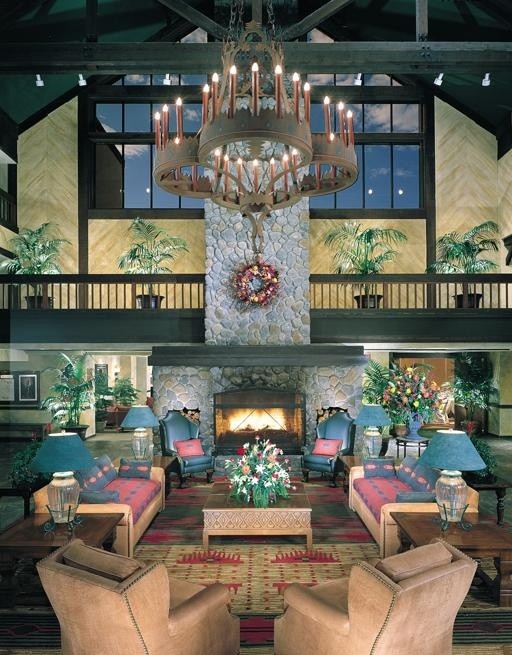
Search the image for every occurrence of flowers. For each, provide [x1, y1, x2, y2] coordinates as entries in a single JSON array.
[[224, 435, 297, 509]]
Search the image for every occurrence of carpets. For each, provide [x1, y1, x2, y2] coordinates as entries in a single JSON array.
[[0, 477, 511, 655]]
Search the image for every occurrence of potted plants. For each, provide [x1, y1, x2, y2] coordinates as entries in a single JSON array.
[[361, 352, 498, 457], [39, 350, 142, 440]]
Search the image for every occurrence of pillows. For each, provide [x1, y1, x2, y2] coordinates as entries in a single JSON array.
[[74, 454, 152, 504], [174, 438, 204, 457], [312, 439, 342, 456], [363, 454, 441, 503]]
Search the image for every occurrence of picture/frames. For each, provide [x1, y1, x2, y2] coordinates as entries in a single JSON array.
[[0, 370, 38, 403]]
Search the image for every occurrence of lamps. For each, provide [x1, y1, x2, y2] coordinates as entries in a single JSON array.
[[30, 430, 96, 533], [416, 428, 487, 532], [120, 405, 160, 459]]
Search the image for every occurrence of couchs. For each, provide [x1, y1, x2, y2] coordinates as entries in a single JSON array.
[[349, 454, 480, 559], [33, 454, 166, 557]]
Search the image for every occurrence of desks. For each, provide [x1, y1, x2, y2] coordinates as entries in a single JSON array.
[[0, 423, 50, 442]]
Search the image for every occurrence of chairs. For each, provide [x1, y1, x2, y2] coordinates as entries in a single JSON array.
[[300, 412, 356, 488], [159, 410, 216, 488]]
